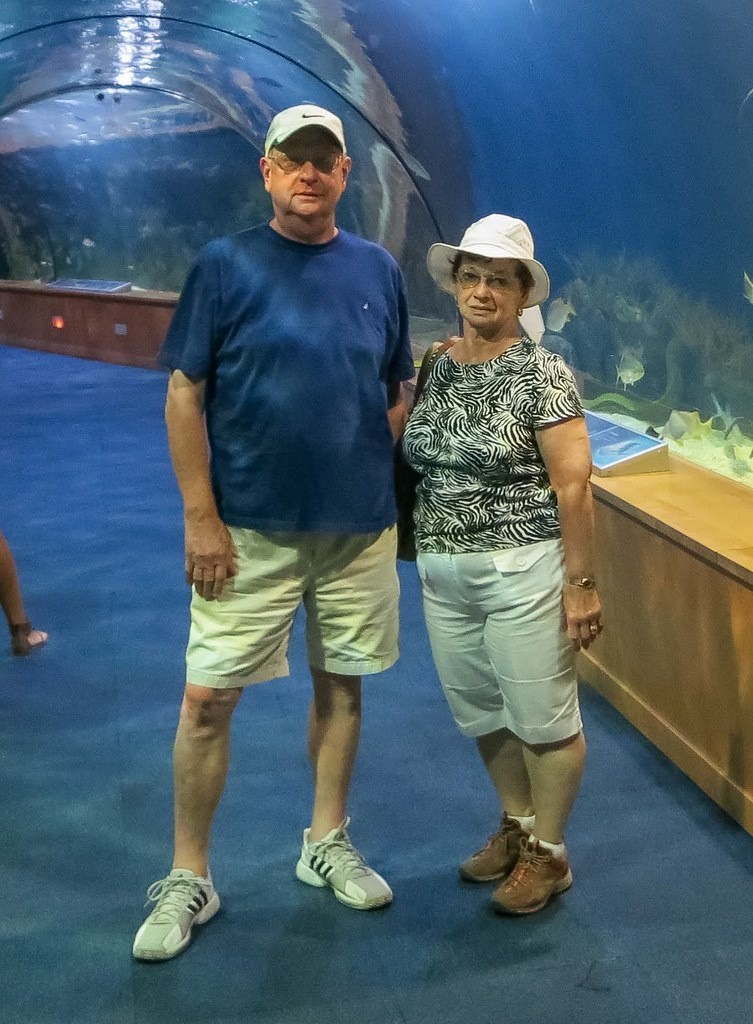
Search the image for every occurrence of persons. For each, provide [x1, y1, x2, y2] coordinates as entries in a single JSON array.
[[0, 534, 48, 656], [132, 104, 415, 961], [398, 214, 604, 914]]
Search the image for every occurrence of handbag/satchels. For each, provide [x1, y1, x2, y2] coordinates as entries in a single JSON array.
[[393, 447, 424, 562]]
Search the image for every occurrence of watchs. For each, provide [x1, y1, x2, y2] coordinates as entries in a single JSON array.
[[564, 575, 596, 590]]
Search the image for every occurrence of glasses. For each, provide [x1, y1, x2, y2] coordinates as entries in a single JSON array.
[[454, 265, 522, 291], [268, 151, 341, 172]]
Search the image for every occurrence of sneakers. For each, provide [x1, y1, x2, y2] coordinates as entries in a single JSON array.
[[459, 810, 531, 882], [133, 864, 220, 961], [490, 837, 573, 913], [296, 817, 393, 910]]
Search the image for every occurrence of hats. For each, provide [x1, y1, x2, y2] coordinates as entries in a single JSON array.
[[426, 214, 550, 309], [265, 104, 347, 158]]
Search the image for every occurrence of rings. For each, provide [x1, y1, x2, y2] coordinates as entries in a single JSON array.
[[590, 625, 598, 631]]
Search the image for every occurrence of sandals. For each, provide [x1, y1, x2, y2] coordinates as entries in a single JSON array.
[[8, 620, 48, 655]]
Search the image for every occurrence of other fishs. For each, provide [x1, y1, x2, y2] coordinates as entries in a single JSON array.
[[545, 297, 577, 334], [615, 352, 646, 391], [253, 75, 283, 88], [662, 410, 753, 478], [743, 270, 753, 306]]
